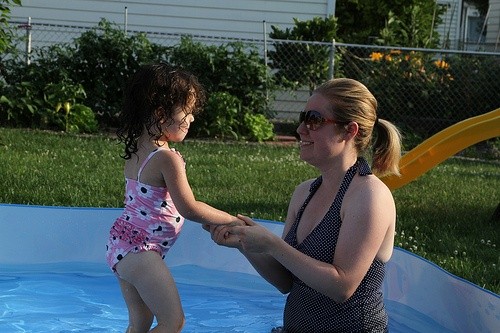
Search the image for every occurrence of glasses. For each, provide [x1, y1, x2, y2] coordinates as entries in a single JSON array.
[[300, 110, 362, 137]]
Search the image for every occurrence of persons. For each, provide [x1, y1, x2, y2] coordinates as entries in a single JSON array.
[[205, 76, 407, 333], [105, 63, 246, 333]]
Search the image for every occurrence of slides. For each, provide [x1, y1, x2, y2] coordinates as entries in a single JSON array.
[[374, 108, 499, 193]]
